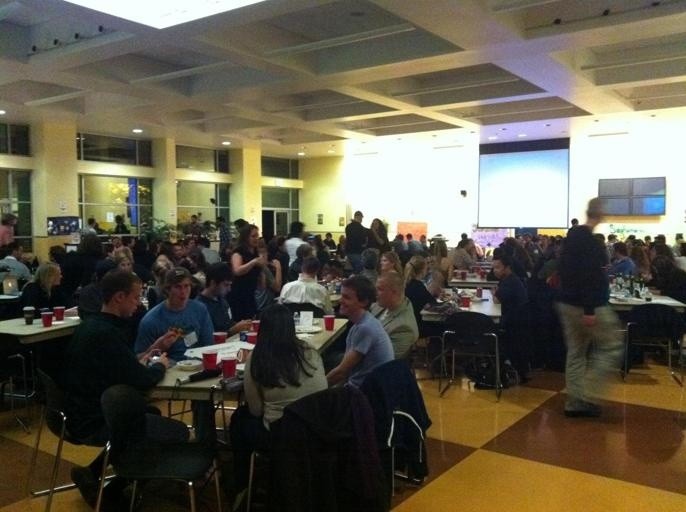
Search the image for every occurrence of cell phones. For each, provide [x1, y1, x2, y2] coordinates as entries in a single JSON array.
[[147, 354, 160, 368]]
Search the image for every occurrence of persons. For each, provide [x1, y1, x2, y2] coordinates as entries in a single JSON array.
[[554, 198, 624, 415], [487, 219, 563, 385], [595, 232, 685, 374], [43, 261, 420, 512], [2, 211, 483, 394]]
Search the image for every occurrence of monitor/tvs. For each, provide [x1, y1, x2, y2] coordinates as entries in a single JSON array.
[[47, 216, 79, 235]]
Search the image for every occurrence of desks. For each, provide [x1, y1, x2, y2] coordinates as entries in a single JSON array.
[[142, 315, 348, 441], [1, 308, 96, 402]]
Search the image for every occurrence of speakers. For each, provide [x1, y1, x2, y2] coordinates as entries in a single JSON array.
[[632, 178, 665, 197], [599, 198, 632, 216], [632, 196, 665, 216], [599, 179, 632, 197]]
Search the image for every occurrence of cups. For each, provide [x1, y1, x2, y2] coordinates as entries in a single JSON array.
[[251, 320, 260, 332], [245, 332, 257, 343], [39, 307, 49, 313], [324, 279, 346, 294], [22, 306, 35, 325], [608, 274, 614, 283], [623, 274, 632, 289], [221, 356, 236, 378], [11, 288, 19, 296], [201, 349, 217, 369], [150, 348, 164, 365], [451, 269, 486, 307], [322, 315, 336, 331], [213, 332, 227, 344], [40, 312, 54, 327], [52, 306, 66, 320]]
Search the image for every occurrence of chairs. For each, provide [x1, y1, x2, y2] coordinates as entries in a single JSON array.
[[365, 355, 424, 502], [0, 344, 44, 433], [28, 368, 108, 510], [255, 386, 378, 511], [621, 302, 685, 387], [98, 387, 223, 509], [436, 311, 521, 399]]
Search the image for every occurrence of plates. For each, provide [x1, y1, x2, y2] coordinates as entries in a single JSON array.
[[234, 362, 247, 372], [176, 360, 201, 371], [294, 326, 321, 334]]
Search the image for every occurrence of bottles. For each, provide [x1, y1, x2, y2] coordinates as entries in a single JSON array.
[[633, 272, 652, 301], [292, 312, 298, 325], [615, 272, 622, 290]]
